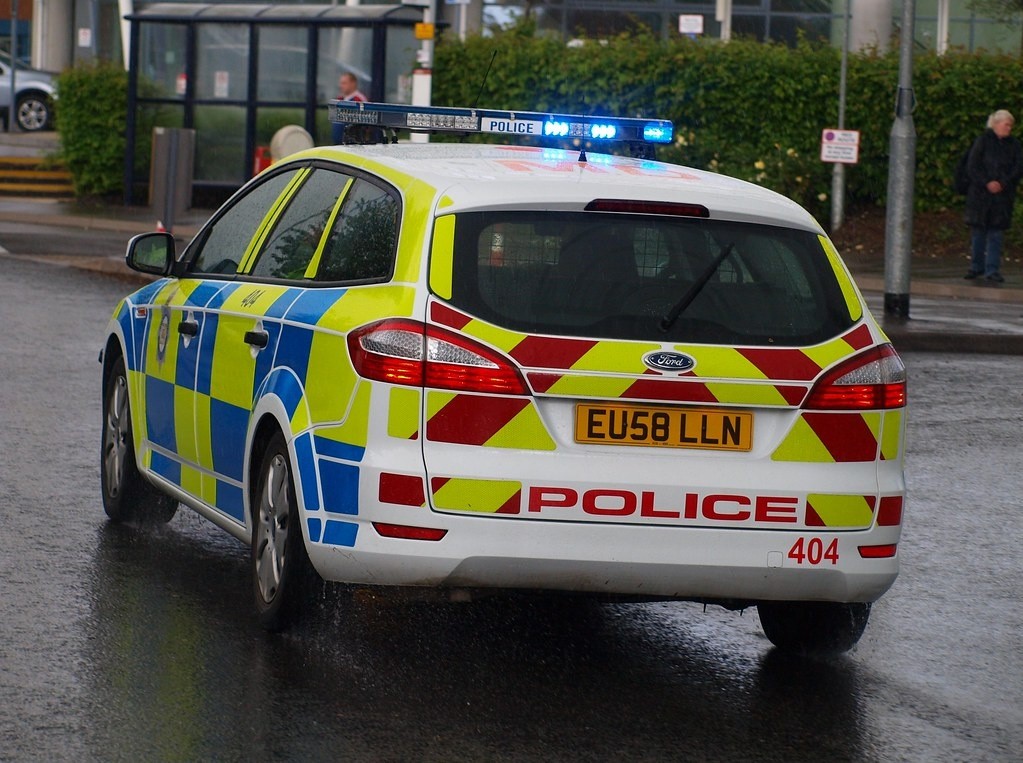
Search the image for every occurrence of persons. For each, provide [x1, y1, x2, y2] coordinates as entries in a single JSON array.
[[963, 109, 1020, 283], [332, 73, 378, 145]]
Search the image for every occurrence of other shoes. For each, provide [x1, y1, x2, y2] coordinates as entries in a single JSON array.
[[963, 268, 984, 280], [987, 272, 1004, 282]]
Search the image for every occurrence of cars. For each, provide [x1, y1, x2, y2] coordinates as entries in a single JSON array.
[[101, 101, 909, 666], [0, 50, 62, 134]]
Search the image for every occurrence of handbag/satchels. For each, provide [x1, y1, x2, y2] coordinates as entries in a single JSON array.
[[952, 158, 975, 194]]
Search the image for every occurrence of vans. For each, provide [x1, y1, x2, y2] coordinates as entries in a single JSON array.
[[173, 45, 373, 105]]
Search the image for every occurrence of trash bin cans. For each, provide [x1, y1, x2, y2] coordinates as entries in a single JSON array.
[[149, 126, 196, 232]]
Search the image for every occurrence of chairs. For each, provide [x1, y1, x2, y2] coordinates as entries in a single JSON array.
[[550, 224, 641, 316]]
